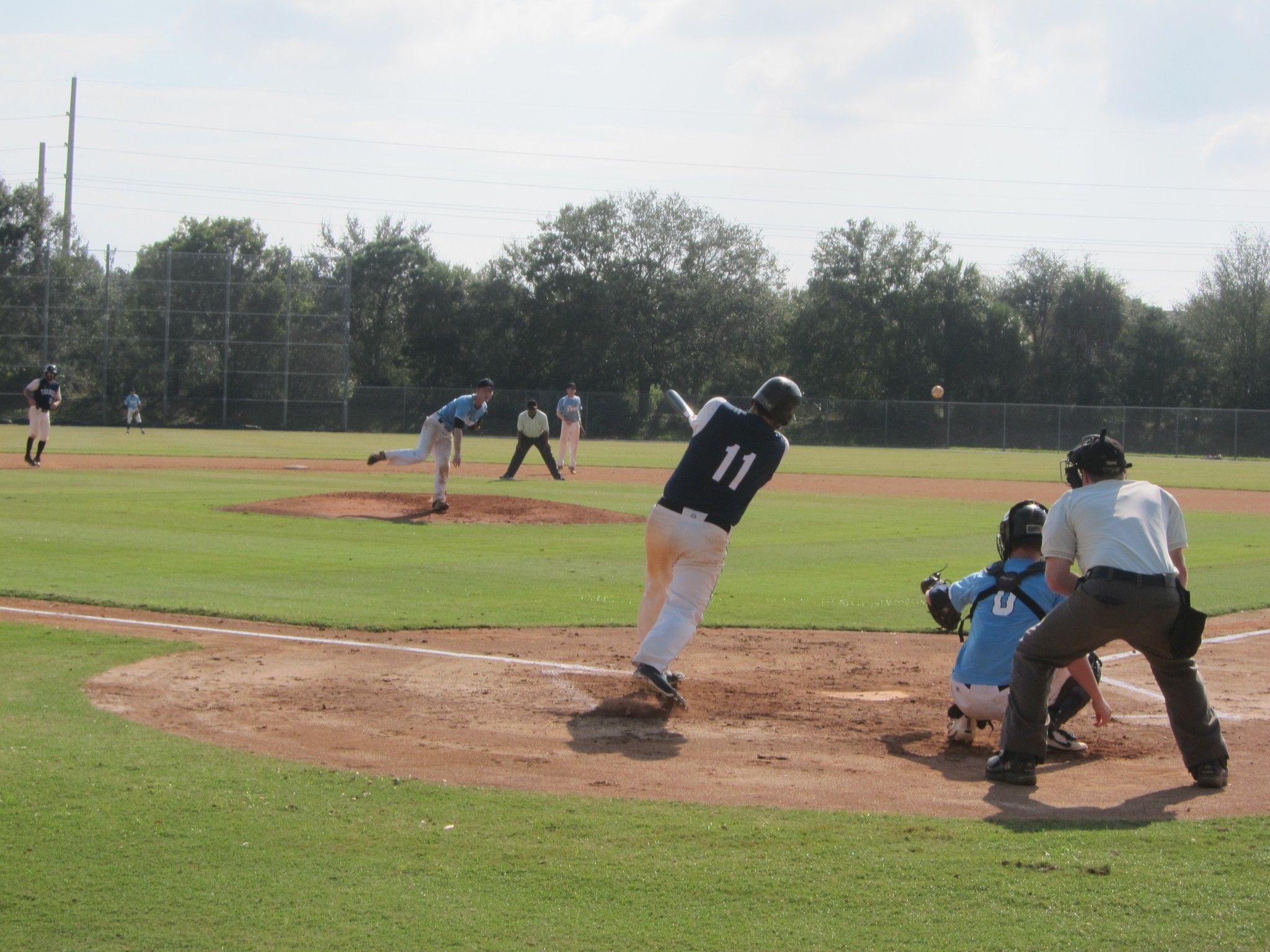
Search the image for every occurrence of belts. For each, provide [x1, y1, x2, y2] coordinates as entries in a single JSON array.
[[36, 406, 49, 414], [438, 418, 451, 432], [1085, 565, 1177, 588], [964, 684, 1009, 691], [658, 498, 731, 533]]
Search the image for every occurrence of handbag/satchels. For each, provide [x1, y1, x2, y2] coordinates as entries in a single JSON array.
[[1169, 578, 1208, 659]]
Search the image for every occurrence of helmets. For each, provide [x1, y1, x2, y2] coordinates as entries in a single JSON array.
[[752, 376, 803, 420], [996, 499, 1049, 562], [44, 364, 57, 375], [1065, 428, 1132, 490]]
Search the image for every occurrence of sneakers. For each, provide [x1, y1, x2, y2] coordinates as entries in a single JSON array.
[[367, 449, 384, 465], [986, 756, 1037, 785], [664, 669, 684, 684], [632, 663, 689, 712], [1197, 762, 1228, 787], [947, 714, 977, 744], [433, 500, 449, 512], [1047, 729, 1088, 751]]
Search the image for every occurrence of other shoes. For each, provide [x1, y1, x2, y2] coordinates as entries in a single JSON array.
[[33, 457, 40, 467], [499, 475, 513, 479], [568, 466, 576, 474], [25, 456, 35, 466], [555, 476, 566, 480], [558, 465, 563, 474]]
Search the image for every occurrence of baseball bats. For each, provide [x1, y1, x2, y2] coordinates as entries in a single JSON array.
[[664, 389, 694, 419]]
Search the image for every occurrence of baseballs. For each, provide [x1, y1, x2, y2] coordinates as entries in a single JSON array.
[[932, 386, 944, 398]]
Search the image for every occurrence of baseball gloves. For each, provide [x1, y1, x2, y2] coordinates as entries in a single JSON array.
[[468, 410, 488, 431], [920, 577, 961, 630], [579, 426, 586, 439]]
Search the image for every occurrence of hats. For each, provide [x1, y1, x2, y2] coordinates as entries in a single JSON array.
[[567, 382, 576, 388], [527, 400, 537, 409], [478, 378, 495, 389]]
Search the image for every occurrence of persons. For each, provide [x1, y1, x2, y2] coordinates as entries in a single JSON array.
[[556, 382, 582, 474], [24, 364, 62, 466], [631, 377, 802, 697], [499, 400, 566, 480], [986, 426, 1230, 788], [368, 378, 494, 510], [920, 500, 1110, 750], [125, 388, 145, 434]]
